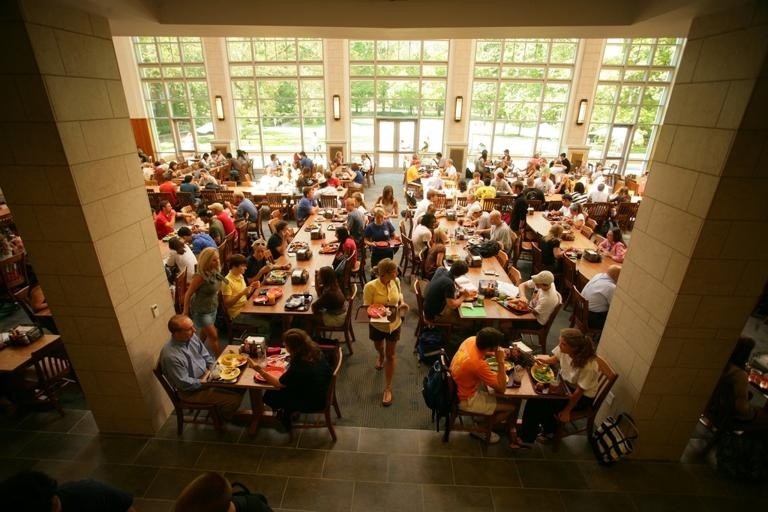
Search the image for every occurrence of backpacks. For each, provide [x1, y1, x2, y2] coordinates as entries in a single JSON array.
[[421, 360, 457, 416]]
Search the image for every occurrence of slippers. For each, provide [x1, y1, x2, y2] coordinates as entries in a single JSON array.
[[509, 437, 533, 448]]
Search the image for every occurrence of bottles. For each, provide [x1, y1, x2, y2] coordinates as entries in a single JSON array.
[[512, 343, 518, 362], [535, 382, 544, 394], [265, 346, 282, 355], [8, 330, 30, 344], [486, 282, 495, 298], [241, 340, 261, 357]]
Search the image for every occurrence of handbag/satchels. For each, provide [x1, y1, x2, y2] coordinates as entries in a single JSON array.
[[445, 335, 467, 364], [419, 329, 445, 366], [591, 412, 640, 466], [463, 241, 499, 257]]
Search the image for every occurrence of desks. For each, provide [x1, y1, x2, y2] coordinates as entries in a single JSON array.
[[200, 345, 294, 429], [489, 354, 572, 440], [1, 329, 61, 393], [508, 177, 528, 186], [158, 216, 207, 268]]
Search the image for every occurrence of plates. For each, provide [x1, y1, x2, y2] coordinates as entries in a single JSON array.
[[490, 361, 514, 372], [377, 239, 402, 247], [367, 304, 386, 318], [210, 353, 247, 381], [163, 217, 208, 242], [265, 211, 346, 284], [456, 290, 478, 302], [255, 354, 289, 382], [251, 295, 268, 303], [267, 287, 282, 298], [506, 299, 530, 312], [286, 299, 301, 308], [531, 363, 554, 385], [434, 207, 485, 265]]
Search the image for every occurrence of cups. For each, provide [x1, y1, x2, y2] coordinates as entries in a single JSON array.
[[499, 292, 505, 302], [512, 368, 525, 385], [303, 289, 310, 299], [547, 377, 560, 392], [577, 252, 582, 260], [255, 281, 260, 288], [269, 293, 275, 303], [258, 350, 267, 368], [477, 295, 484, 305], [259, 290, 266, 295]]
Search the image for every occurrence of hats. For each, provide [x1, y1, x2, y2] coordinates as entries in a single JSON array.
[[469, 204, 481, 213], [531, 270, 555, 284], [207, 203, 223, 212], [169, 471, 232, 512]]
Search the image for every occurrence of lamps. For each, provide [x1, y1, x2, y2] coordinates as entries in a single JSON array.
[[576, 99, 588, 125], [453, 96, 463, 121], [215, 95, 224, 120], [332, 94, 340, 120]]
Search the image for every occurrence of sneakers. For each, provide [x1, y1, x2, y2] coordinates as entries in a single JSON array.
[[470, 423, 500, 443], [376, 354, 384, 369], [382, 386, 393, 406]]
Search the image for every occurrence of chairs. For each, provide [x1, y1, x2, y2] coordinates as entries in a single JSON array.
[[221, 204, 373, 346], [1, 252, 44, 327], [439, 347, 496, 449], [405, 158, 461, 199], [625, 174, 639, 195], [309, 159, 376, 208], [144, 179, 354, 219], [575, 161, 617, 185], [153, 361, 227, 445], [171, 154, 253, 179], [288, 347, 344, 441], [174, 204, 265, 314], [30, 338, 82, 419], [545, 353, 620, 451], [399, 195, 639, 368], [702, 377, 756, 457]]
